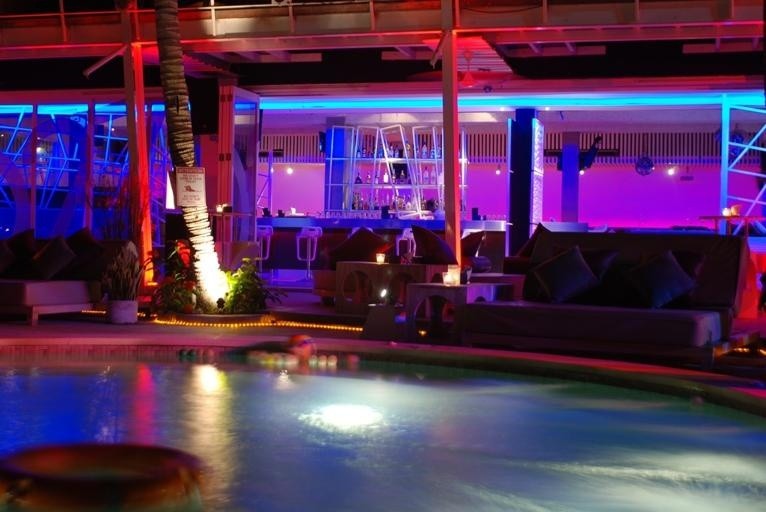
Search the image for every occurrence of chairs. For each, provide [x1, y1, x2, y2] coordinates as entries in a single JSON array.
[[351, 226, 373, 235], [254, 224, 273, 280], [396, 228, 416, 257], [296, 226, 322, 281]]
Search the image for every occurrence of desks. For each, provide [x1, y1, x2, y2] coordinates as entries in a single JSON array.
[[334, 261, 448, 313], [469, 273, 527, 305], [406, 282, 493, 342]]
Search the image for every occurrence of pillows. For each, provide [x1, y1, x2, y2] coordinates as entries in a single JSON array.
[[620, 247, 700, 311], [32, 232, 76, 279], [64, 227, 100, 269], [324, 226, 395, 270], [411, 225, 458, 264], [519, 223, 618, 304], [0, 228, 38, 276]]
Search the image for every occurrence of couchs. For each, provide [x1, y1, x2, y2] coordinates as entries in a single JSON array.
[[1, 240, 136, 327], [487, 230, 749, 369], [313, 229, 448, 306]]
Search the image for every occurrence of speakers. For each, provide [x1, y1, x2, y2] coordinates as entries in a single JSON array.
[[191, 78, 219, 135]]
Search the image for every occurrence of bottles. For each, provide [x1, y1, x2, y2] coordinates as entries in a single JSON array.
[[351, 140, 444, 210]]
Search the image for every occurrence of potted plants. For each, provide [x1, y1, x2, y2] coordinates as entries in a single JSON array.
[[100, 244, 139, 324]]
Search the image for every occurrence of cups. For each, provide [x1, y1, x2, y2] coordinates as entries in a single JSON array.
[[731, 206, 739, 216], [485, 214, 508, 221], [442, 264, 462, 287], [721, 207, 732, 216], [375, 253, 385, 263], [262, 207, 286, 217], [303, 209, 379, 218]]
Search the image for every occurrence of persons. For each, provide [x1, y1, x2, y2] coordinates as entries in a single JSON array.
[[424, 198, 445, 220]]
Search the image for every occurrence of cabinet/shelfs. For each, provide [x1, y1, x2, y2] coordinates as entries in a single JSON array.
[[324, 157, 470, 216]]
